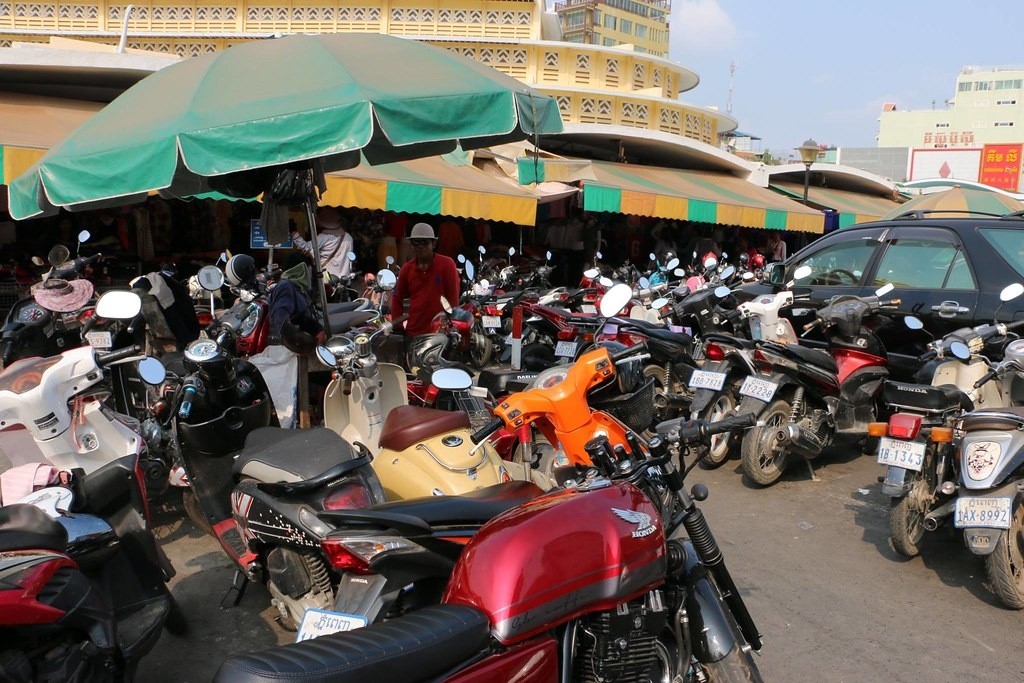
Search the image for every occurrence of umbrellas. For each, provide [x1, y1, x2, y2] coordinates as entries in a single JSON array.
[[878, 183, 1024, 220], [9, 31, 568, 431]]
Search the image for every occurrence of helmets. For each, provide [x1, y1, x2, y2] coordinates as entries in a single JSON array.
[[751, 254, 766, 268], [582, 340, 641, 392], [11, 486, 120, 572], [226, 254, 256, 285], [280, 311, 326, 355], [701, 250, 719, 271], [406, 333, 475, 383]]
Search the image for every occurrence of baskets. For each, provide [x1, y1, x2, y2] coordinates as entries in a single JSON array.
[[588, 375, 655, 435]]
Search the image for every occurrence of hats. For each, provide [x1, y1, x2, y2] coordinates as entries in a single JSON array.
[[405, 222, 439, 239], [316, 207, 342, 228], [31, 278, 93, 311]]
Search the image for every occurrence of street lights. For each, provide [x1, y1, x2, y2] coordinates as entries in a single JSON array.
[[793, 138, 824, 250]]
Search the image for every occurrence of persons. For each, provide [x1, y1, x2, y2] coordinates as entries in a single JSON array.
[[290, 205, 356, 303], [266, 248, 328, 428], [391, 222, 462, 380], [358, 209, 790, 292]]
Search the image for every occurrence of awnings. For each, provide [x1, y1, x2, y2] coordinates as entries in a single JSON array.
[[1, 87, 539, 228], [767, 178, 904, 231], [558, 153, 826, 236], [437, 128, 599, 186]]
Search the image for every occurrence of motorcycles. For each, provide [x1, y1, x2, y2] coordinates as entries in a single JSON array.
[[0, 217, 1023, 683]]
